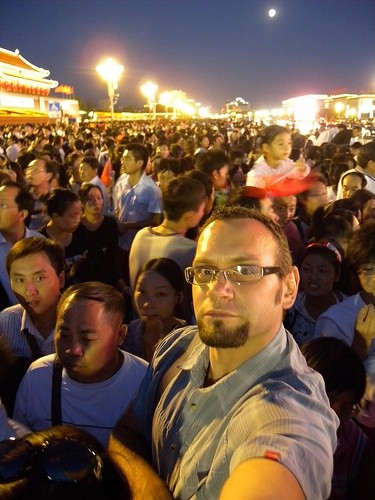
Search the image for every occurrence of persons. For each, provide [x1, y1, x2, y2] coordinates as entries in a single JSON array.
[[111, 144, 163, 224], [13, 280, 149, 440], [0, 119, 375, 500], [110, 205, 341, 500], [245, 125, 311, 206]]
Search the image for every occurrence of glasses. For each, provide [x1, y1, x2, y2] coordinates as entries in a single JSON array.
[[185, 264, 283, 286], [358, 267, 375, 279], [120, 157, 138, 162]]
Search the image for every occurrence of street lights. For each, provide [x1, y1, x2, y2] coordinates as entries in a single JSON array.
[[139, 81, 158, 123], [94, 56, 126, 130], [159, 89, 209, 120]]
